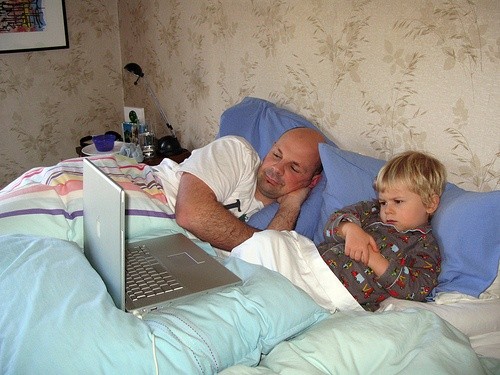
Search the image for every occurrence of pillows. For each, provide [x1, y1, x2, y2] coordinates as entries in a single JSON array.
[[216, 97, 341, 238], [313, 141, 500, 297]]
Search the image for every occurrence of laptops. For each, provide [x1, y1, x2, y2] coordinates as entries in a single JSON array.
[[82, 158, 243, 314]]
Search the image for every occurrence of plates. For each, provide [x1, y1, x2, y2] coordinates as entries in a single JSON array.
[[81, 141, 125, 156]]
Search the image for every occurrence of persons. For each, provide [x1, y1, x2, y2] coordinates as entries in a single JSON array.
[[315, 151, 447, 312], [150, 127, 326, 253]]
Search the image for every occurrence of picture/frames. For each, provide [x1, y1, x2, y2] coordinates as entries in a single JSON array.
[[0, 0, 69, 53]]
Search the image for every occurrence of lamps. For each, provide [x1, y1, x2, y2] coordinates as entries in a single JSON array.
[[124, 63, 182, 153]]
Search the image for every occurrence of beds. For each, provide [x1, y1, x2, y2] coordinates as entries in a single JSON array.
[[0, 155, 500, 374]]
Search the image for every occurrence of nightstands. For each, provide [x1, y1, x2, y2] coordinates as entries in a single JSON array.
[[76, 145, 192, 166]]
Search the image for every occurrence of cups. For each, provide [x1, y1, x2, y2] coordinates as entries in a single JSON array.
[[92, 134, 116, 152], [123, 122, 138, 146], [137, 118, 155, 158]]
